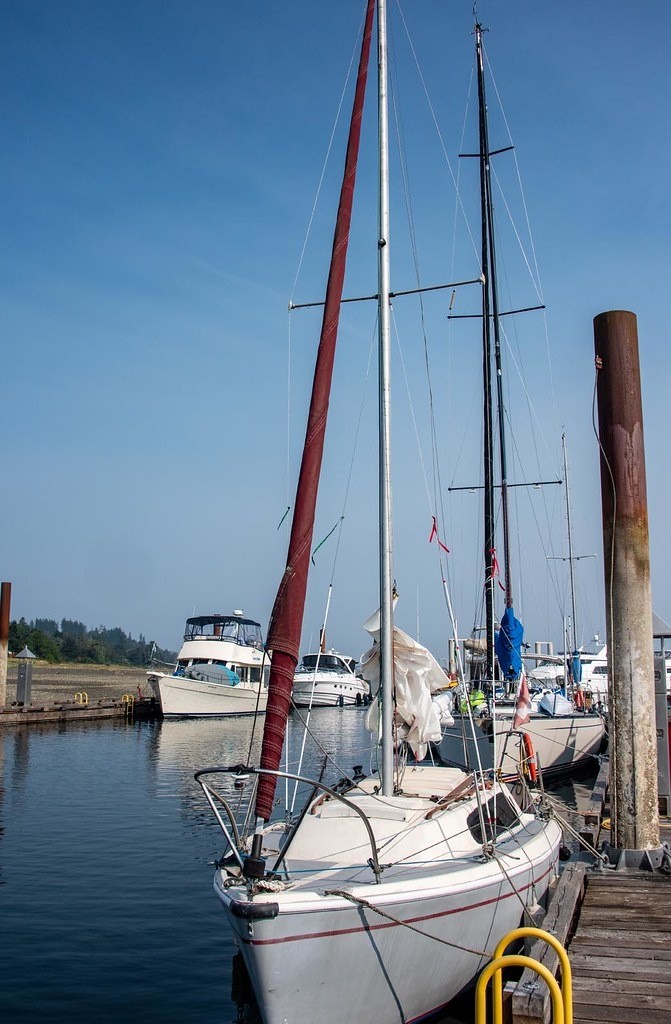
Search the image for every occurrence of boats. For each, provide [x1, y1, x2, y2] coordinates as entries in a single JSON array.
[[526, 634, 609, 714], [146, 609, 272, 719], [292, 624, 371, 708]]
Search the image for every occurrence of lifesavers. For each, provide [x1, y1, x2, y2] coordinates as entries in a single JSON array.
[[575, 690, 583, 708], [520, 732, 537, 783]]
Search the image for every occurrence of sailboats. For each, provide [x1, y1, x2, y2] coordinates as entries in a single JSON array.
[[431, 2, 607, 788], [194, 0, 561, 1024]]
